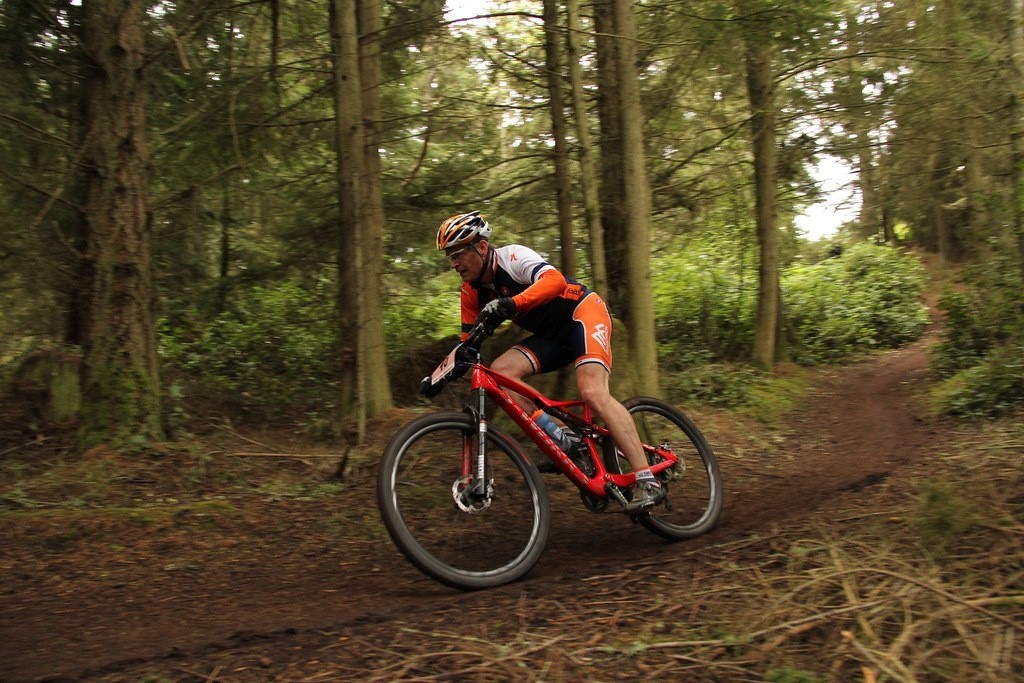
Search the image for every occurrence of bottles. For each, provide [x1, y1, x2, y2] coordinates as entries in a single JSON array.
[[531, 409, 572, 452]]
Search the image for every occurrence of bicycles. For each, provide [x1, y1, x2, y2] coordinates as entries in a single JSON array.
[[376, 305, 723, 593]]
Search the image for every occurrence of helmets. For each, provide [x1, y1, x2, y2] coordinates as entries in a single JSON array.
[[436, 209, 493, 251]]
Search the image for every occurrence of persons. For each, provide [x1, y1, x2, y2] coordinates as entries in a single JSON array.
[[419, 210, 667, 512]]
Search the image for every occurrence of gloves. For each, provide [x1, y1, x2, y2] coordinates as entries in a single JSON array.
[[419, 375, 444, 399], [477, 296, 517, 337]]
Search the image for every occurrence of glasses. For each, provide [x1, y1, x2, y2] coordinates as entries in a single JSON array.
[[446, 241, 476, 262]]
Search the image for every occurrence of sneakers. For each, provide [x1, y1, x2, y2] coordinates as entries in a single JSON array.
[[626, 479, 667, 511]]
